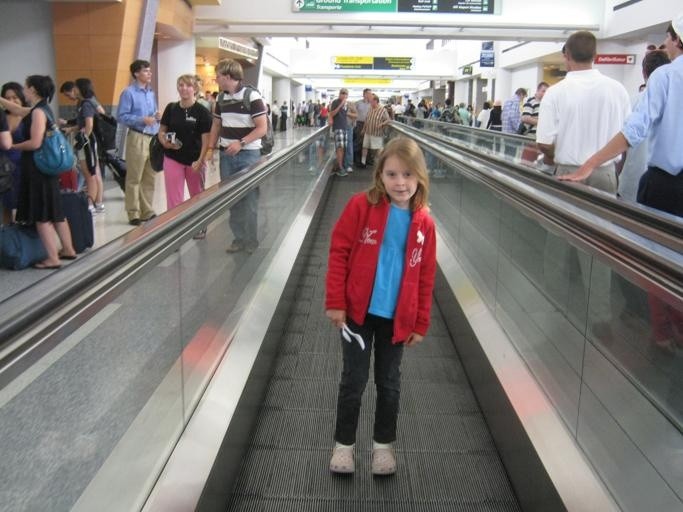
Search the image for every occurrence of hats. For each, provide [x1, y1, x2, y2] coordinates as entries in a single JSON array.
[[671, 13, 683, 42], [493, 99, 502, 107]]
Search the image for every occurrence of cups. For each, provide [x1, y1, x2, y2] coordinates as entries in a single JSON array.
[[166, 132, 176, 145]]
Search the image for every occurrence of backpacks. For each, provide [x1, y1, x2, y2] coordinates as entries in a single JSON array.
[[0, 223, 49, 271], [218, 85, 274, 156]]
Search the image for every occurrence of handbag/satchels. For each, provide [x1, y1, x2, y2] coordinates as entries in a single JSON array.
[[30, 130, 77, 174], [149, 132, 163, 172], [83, 113, 117, 151]]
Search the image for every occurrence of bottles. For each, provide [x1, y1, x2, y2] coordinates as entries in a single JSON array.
[[532, 154, 546, 169]]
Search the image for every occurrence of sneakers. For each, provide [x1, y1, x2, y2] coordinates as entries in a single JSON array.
[[88, 202, 105, 216], [193, 227, 206, 238], [334, 163, 366, 177], [226, 241, 258, 255], [128, 219, 140, 225]]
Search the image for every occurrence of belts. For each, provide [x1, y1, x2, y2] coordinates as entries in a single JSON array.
[[130, 127, 154, 137]]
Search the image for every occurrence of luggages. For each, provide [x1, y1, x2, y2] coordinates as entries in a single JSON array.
[[57, 172, 93, 254], [102, 152, 126, 192]]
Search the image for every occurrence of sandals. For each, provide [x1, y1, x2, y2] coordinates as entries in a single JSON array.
[[370, 440, 395, 475], [328, 443, 355, 474]]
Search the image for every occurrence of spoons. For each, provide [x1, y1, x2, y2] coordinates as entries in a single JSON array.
[[341, 326, 351, 343], [343, 323, 366, 351]]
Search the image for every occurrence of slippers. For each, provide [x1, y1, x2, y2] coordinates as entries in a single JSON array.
[[34, 262, 62, 271], [58, 252, 76, 260]]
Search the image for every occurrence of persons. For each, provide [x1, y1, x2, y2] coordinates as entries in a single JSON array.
[[324, 136, 436, 477]]
